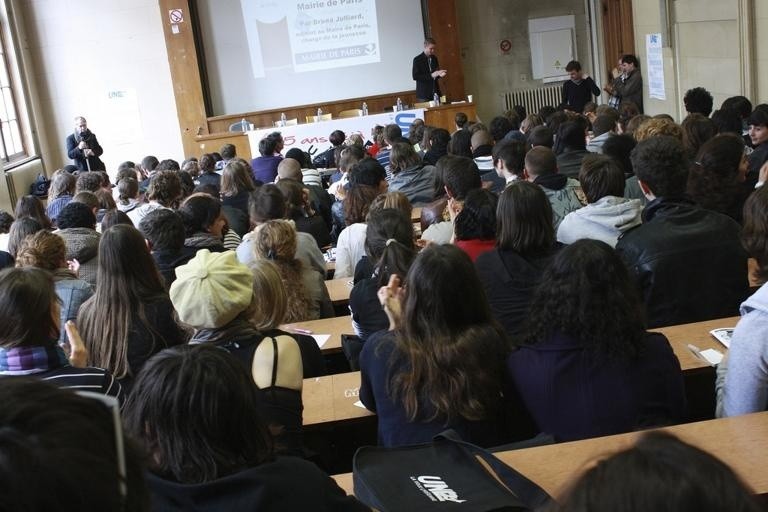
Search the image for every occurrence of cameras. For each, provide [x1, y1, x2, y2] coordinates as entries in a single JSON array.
[[79, 131, 88, 137]]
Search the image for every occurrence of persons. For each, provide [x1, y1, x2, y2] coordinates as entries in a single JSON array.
[[562, 61, 600, 113], [603, 54, 629, 109], [413, 38, 448, 102], [612, 56, 642, 114]]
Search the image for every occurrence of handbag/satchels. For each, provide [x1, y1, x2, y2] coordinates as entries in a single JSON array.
[[351, 429, 563, 511]]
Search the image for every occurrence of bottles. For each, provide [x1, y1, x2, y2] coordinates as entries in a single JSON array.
[[433, 93, 440, 107], [248, 123, 255, 130], [397, 98, 402, 112], [241, 117, 246, 133], [281, 113, 287, 128], [318, 108, 322, 122], [363, 101, 368, 116]]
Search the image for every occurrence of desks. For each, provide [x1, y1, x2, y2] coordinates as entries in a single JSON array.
[[80, 198, 768, 512]]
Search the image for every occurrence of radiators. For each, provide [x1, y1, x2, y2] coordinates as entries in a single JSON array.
[[501, 85, 565, 120], [2, 154, 50, 216]]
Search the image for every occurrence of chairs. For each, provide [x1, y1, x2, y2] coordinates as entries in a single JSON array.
[[229, 98, 440, 131]]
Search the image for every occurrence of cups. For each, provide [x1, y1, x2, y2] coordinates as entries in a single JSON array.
[[313, 116, 317, 123], [467, 95, 472, 104], [429, 101, 435, 107], [393, 106, 398, 112], [359, 110, 363, 116]]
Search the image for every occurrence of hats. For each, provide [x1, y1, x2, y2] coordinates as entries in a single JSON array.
[[168, 248, 255, 329]]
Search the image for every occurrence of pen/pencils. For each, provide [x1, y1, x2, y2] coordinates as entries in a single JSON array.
[[293, 329, 313, 334]]
